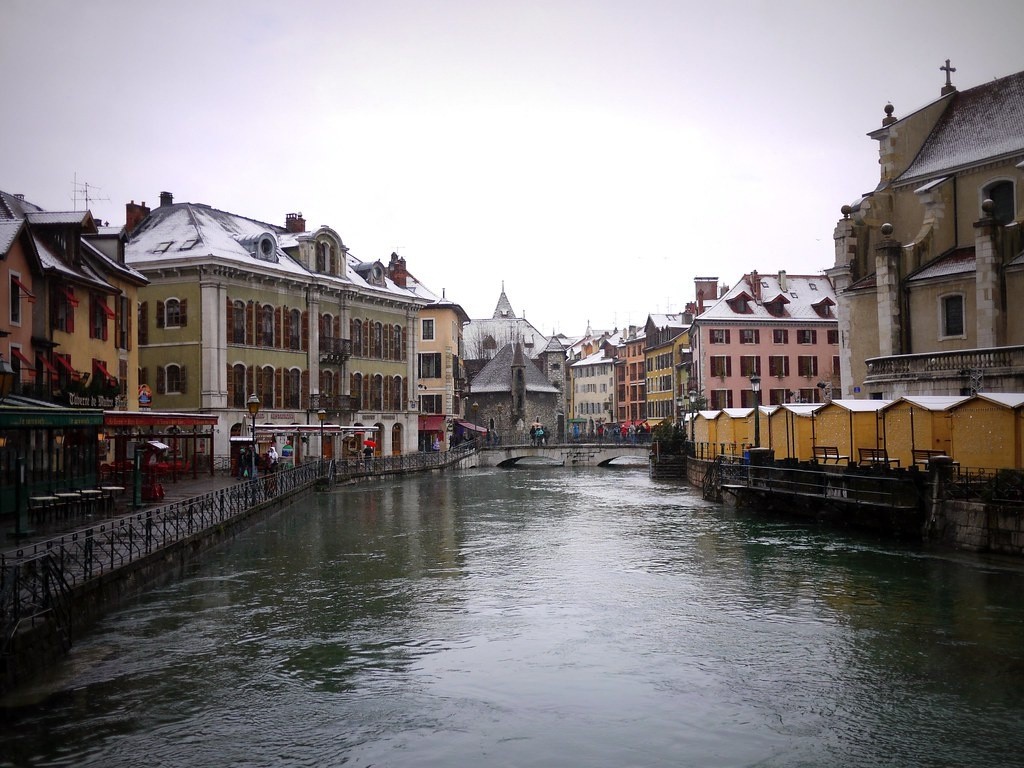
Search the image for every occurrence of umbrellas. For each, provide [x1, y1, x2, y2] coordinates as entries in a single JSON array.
[[363, 440, 376, 447]]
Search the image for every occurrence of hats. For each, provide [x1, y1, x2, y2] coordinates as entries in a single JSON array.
[[537, 427, 541, 429], [536, 425, 539, 426], [532, 425, 535, 427]]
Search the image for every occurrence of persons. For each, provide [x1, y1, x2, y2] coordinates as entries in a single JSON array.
[[530, 425, 550, 446], [449, 429, 498, 451], [363, 445, 374, 470], [596, 422, 652, 443], [236, 445, 278, 481]]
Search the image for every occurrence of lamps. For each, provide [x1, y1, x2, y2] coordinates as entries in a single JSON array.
[[83, 372, 91, 379]]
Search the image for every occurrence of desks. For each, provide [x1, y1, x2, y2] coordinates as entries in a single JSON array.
[[29, 486, 125, 535]]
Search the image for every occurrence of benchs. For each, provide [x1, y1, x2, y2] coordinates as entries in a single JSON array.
[[858, 448, 900, 467], [911, 449, 961, 474], [813, 446, 849, 464]]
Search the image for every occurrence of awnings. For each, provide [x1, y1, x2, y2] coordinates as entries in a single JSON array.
[[94, 298, 116, 320], [55, 355, 80, 381], [37, 353, 59, 381], [57, 286, 80, 308], [12, 349, 38, 376], [458, 422, 491, 433], [11, 277, 37, 304], [96, 364, 116, 387], [620, 419, 663, 428]]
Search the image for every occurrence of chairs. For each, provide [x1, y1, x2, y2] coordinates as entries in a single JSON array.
[[101, 460, 190, 482]]
